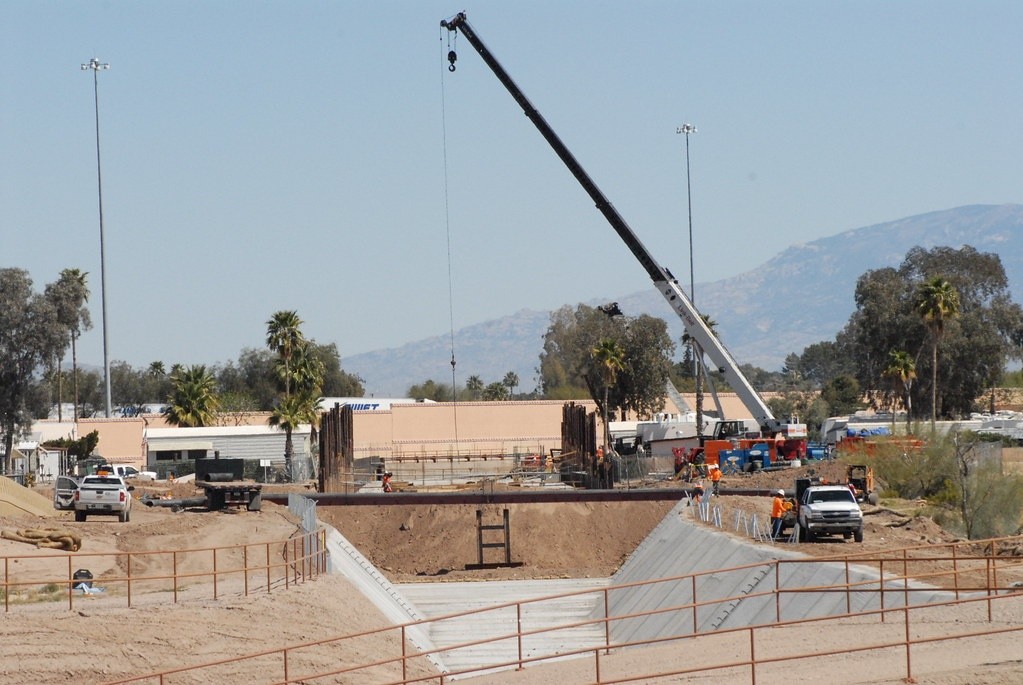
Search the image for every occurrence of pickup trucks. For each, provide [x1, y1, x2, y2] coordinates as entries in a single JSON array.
[[54, 474, 136, 523]]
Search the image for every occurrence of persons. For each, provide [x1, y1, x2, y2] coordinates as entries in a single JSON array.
[[687, 486, 705, 506], [709, 464, 722, 497], [383, 471, 392, 492], [772, 489, 787, 538]]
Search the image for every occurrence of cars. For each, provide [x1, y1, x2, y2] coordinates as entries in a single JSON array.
[[94, 463, 144, 479], [791, 484, 864, 543]]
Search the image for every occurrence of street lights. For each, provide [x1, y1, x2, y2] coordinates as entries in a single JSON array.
[[678, 120, 698, 380], [81, 56, 114, 417]]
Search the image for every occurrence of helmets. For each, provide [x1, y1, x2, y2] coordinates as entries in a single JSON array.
[[714, 464, 719, 468], [777, 489, 786, 496]]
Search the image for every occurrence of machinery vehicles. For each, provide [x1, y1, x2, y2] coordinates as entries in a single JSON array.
[[438, 11, 805, 474]]
